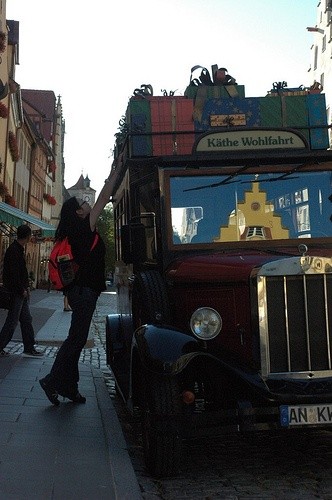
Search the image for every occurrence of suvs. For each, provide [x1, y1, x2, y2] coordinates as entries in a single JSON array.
[[104, 160, 332, 470]]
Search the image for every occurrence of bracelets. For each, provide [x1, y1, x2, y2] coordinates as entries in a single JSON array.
[[104, 179, 110, 183]]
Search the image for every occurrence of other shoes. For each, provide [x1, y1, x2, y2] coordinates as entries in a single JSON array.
[[23, 349, 42, 356], [63, 307, 72, 311], [0, 350, 10, 357], [38, 373, 86, 406]]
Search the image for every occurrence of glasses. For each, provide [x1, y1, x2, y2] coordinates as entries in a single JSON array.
[[79, 200, 86, 207]]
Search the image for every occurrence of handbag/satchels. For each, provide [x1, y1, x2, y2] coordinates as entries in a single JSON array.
[[0, 286, 16, 311]]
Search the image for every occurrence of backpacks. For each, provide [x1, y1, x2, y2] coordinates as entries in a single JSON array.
[[45, 231, 98, 296]]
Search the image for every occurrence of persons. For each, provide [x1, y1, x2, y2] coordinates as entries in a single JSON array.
[[0, 225, 45, 359], [64, 295, 72, 311], [39, 152, 123, 407]]
